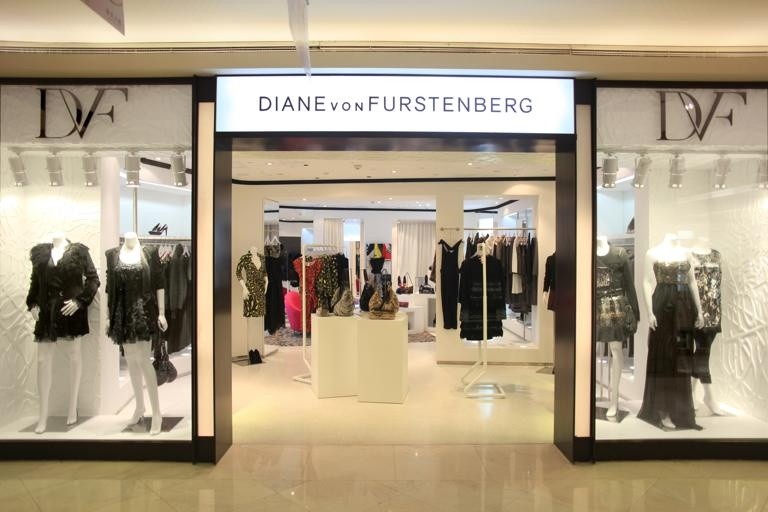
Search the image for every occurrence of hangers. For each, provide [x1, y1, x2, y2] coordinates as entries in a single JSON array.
[[304, 246, 343, 258], [118, 239, 192, 261], [441, 228, 530, 259]]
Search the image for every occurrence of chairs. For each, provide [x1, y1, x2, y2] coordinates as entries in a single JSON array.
[[285, 291, 311, 336]]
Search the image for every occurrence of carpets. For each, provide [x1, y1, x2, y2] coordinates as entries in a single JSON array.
[[263, 325, 436, 346]]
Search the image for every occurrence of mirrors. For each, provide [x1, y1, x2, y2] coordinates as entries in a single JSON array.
[[345, 241, 360, 298], [366, 244, 391, 287]]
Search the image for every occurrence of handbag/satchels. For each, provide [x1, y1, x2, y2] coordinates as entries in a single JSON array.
[[151, 332, 177, 385], [624, 305, 637, 337], [396, 272, 413, 294]]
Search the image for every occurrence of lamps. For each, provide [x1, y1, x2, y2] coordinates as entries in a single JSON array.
[[7, 146, 189, 188], [597, 145, 768, 190]]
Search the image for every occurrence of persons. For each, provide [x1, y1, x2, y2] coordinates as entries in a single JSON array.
[[234, 245, 269, 365], [103, 228, 169, 436], [683, 237, 728, 415], [542, 248, 556, 317], [595, 233, 640, 418], [25, 235, 101, 438], [634, 231, 704, 433]]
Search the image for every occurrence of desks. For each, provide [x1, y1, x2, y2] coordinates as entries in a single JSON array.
[[397, 306, 424, 334], [396, 292, 436, 329]]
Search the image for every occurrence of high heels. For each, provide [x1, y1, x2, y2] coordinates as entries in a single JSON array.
[[249, 349, 262, 363], [148, 223, 168, 235]]
